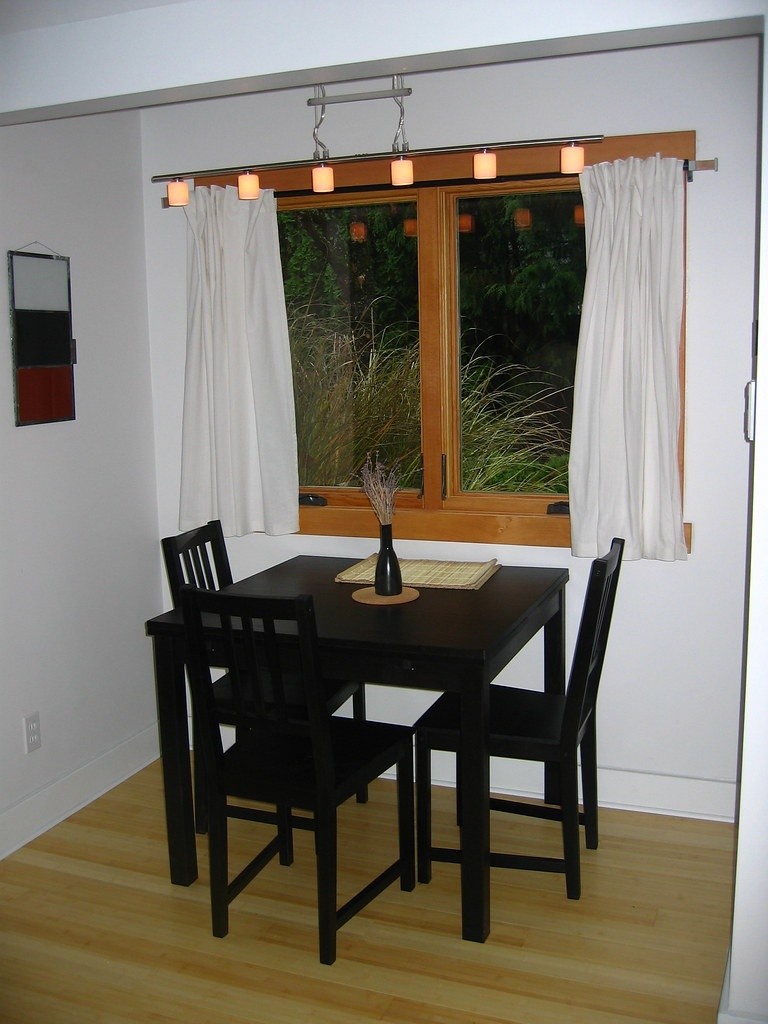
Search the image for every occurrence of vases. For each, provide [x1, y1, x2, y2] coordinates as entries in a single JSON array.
[[374, 523, 402, 597]]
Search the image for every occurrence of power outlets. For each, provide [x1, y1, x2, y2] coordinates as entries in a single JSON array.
[[23, 710, 41, 755]]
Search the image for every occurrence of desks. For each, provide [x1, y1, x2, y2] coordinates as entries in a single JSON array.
[[144, 552, 572, 942]]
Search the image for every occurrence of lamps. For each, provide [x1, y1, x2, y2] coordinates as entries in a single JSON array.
[[151, 74, 604, 205]]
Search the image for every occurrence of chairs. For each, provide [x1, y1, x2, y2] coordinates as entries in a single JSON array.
[[412, 537, 626, 900], [177, 582, 412, 966], [161, 520, 368, 866]]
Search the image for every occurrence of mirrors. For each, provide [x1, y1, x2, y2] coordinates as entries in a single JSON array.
[[8, 250, 76, 427]]
